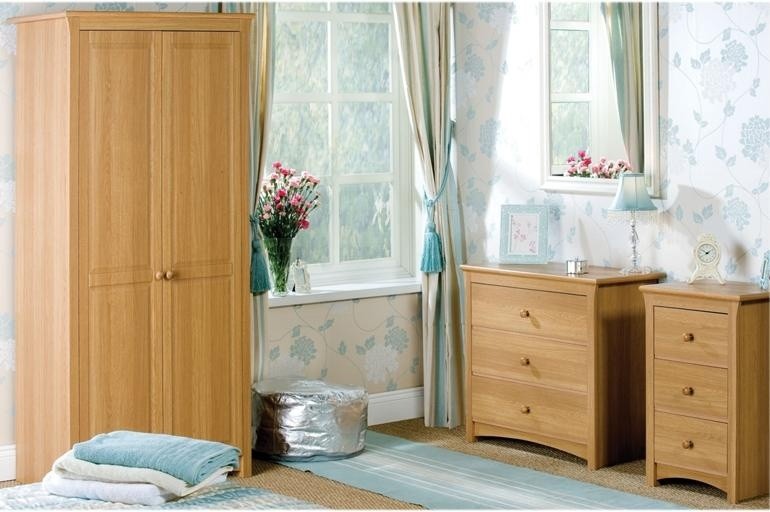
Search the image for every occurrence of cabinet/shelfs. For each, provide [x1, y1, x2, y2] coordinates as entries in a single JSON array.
[[3, 9, 260, 485], [640, 282, 770, 503], [461, 260, 664, 470]]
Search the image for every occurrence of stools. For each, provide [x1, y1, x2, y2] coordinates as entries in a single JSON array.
[[251, 377, 370, 460]]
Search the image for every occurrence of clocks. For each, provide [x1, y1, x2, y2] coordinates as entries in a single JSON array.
[[688, 234, 729, 287]]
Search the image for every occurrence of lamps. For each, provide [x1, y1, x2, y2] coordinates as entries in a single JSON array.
[[604, 171, 661, 275]]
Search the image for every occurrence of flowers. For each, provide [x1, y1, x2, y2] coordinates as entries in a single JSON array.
[[562, 148, 630, 179], [254, 159, 322, 294]]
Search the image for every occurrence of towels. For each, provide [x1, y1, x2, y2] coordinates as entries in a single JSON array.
[[45, 429, 245, 506]]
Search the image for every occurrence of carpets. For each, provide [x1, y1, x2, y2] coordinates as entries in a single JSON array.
[[0, 480, 326, 510], [272, 430, 696, 509]]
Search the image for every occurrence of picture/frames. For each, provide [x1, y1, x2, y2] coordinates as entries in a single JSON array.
[[498, 203, 551, 266]]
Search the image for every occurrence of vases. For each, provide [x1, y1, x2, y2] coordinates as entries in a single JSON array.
[[261, 238, 294, 296]]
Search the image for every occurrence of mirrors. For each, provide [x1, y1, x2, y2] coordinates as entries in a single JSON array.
[[537, 3, 662, 199]]
